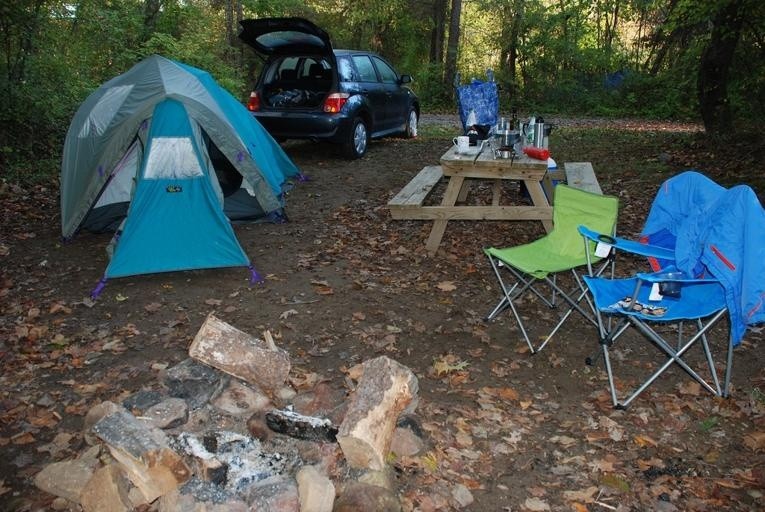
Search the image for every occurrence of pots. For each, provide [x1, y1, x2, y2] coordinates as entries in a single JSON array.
[[493, 129, 521, 146]]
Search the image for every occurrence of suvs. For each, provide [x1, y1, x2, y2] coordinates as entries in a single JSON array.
[[238, 16, 419, 159]]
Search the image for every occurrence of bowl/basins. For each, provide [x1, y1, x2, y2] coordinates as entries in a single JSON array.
[[467, 123, 490, 140]]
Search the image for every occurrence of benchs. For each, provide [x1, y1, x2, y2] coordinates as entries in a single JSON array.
[[387, 165, 442, 207], [564, 162, 602, 195]]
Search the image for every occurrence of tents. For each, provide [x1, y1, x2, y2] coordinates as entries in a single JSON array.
[[59, 51, 303, 298]]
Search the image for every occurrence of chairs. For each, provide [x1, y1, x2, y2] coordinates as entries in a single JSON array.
[[310, 63, 323, 78], [281, 69, 296, 81], [483, 184, 619, 356], [578, 178, 747, 409]]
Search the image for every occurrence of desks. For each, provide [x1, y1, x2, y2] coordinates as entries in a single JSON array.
[[426, 128, 553, 256]]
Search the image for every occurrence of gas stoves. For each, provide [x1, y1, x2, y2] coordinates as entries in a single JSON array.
[[493, 145, 520, 159]]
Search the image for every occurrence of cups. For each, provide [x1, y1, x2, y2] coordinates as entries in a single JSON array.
[[452, 136, 470, 154]]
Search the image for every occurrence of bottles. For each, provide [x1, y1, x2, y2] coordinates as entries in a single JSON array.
[[524, 116, 550, 160], [500, 115, 519, 129]]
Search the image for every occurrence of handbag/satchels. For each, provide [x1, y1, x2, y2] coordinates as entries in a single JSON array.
[[269, 88, 316, 106], [454, 68, 500, 145]]
